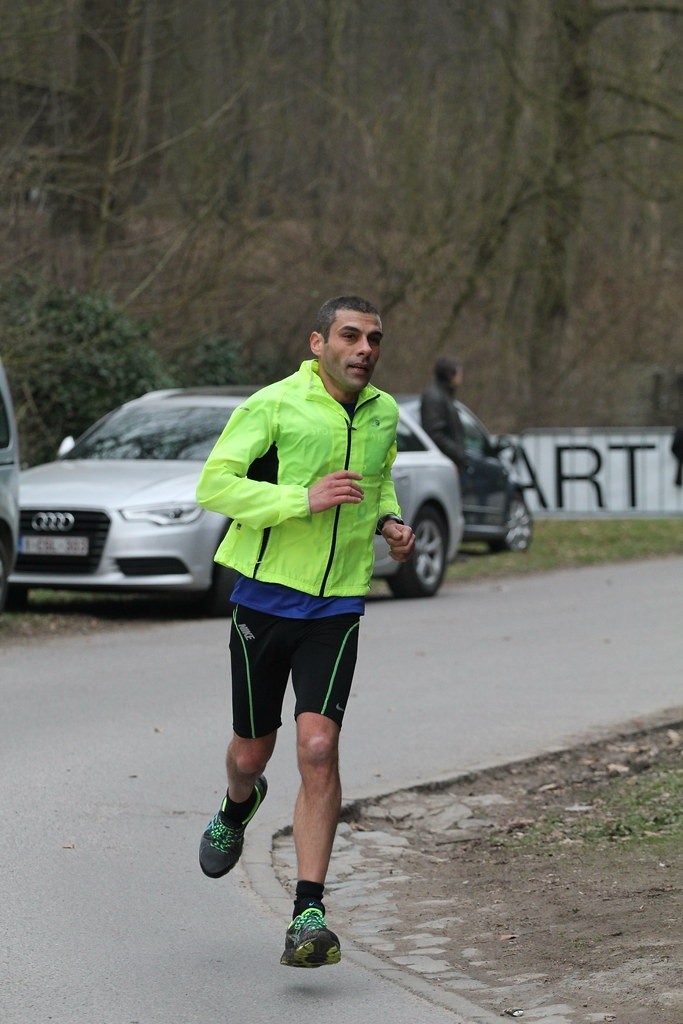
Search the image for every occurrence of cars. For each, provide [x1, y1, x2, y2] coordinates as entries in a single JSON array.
[[0, 356, 25, 623], [382, 390, 535, 555], [16, 384, 468, 620]]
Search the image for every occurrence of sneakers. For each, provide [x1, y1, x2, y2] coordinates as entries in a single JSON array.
[[199, 773, 268, 879], [280, 907, 341, 968]]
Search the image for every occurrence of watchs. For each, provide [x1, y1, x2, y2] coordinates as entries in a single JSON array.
[[375, 513, 405, 535]]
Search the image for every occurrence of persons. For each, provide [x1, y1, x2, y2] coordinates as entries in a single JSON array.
[[418, 358, 470, 500], [196, 294, 416, 967]]
